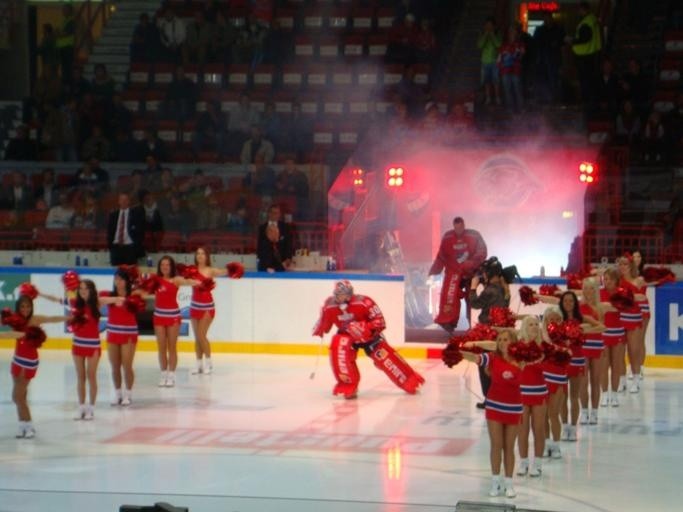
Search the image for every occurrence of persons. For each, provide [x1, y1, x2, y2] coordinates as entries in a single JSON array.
[[488, 291, 605, 441], [0, 172, 32, 212], [567, 249, 677, 408], [340, 11, 475, 142], [475, 16, 503, 110], [227, 92, 263, 164], [566, 251, 683, 394], [467, 257, 511, 409], [447, 314, 573, 477], [41, 96, 82, 163], [275, 100, 313, 164], [73, 155, 112, 187], [158, 168, 177, 198], [74, 91, 106, 118], [89, 63, 117, 115], [159, 193, 196, 234], [1, 295, 87, 439], [176, 246, 244, 375], [77, 191, 104, 231], [34, 167, 61, 209], [270, 158, 309, 209], [134, 124, 169, 164], [539, 268, 631, 408], [240, 125, 275, 188], [106, 190, 146, 265], [196, 96, 229, 153], [106, 94, 134, 142], [121, 255, 216, 387], [137, 188, 165, 245], [199, 194, 229, 232], [117, 0, 195, 64], [75, 126, 115, 163], [45, 194, 75, 229], [260, 102, 280, 151], [523, 3, 683, 170], [441, 328, 545, 498], [257, 204, 295, 272], [202, 0, 271, 67], [125, 169, 145, 201], [63, 269, 145, 406], [229, 198, 252, 231], [519, 276, 634, 425], [16, 280, 145, 421], [427, 218, 487, 331], [473, 307, 572, 459], [495, 21, 526, 119], [141, 153, 163, 191], [4, 126, 46, 161], [312, 282, 425, 399]]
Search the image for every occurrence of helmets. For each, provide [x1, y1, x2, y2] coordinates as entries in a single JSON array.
[[481, 261, 502, 279], [333, 280, 352, 295]]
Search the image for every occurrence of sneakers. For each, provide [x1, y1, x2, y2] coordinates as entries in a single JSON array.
[[24, 425, 36, 438], [15, 426, 25, 438], [542, 444, 551, 457], [488, 483, 501, 496], [600, 396, 609, 407], [551, 444, 561, 458], [588, 414, 598, 424], [165, 377, 175, 387], [629, 383, 640, 393], [203, 363, 213, 375], [84, 410, 94, 419], [611, 397, 619, 407], [191, 364, 203, 374], [110, 395, 122, 406], [568, 429, 576, 441], [503, 483, 515, 498], [72, 410, 85, 420], [618, 381, 628, 392], [121, 395, 132, 405], [477, 402, 486, 408], [560, 428, 568, 440], [415, 383, 425, 394], [639, 368, 643, 379], [579, 413, 589, 424], [337, 392, 358, 399], [157, 378, 166, 387], [529, 461, 542, 477], [627, 370, 633, 379], [516, 462, 529, 475]]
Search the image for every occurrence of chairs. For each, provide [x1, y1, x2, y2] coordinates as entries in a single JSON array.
[[116, 0, 431, 161]]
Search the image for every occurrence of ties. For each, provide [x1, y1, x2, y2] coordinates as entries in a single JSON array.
[[272, 224, 278, 252], [118, 211, 125, 244]]
